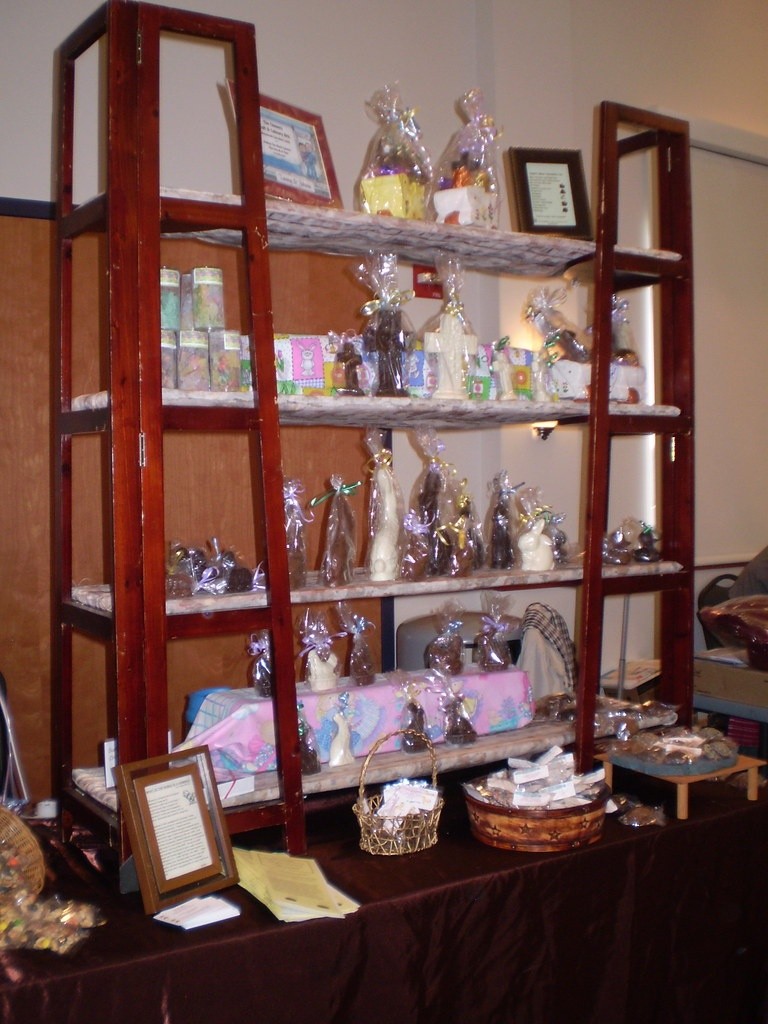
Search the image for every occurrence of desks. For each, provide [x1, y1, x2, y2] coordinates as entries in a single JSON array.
[[0, 770, 768, 1024]]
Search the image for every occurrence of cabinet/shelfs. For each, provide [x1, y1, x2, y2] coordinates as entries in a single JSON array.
[[50, 0, 698, 895]]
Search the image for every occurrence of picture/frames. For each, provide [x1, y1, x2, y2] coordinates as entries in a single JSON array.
[[508, 146, 594, 241], [225, 78, 343, 209]]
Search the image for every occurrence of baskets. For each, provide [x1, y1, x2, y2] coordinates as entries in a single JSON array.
[[351, 728, 445, 855], [0, 810, 44, 920]]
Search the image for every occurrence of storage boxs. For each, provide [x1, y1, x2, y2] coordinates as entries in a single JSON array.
[[693, 647, 768, 707]]
